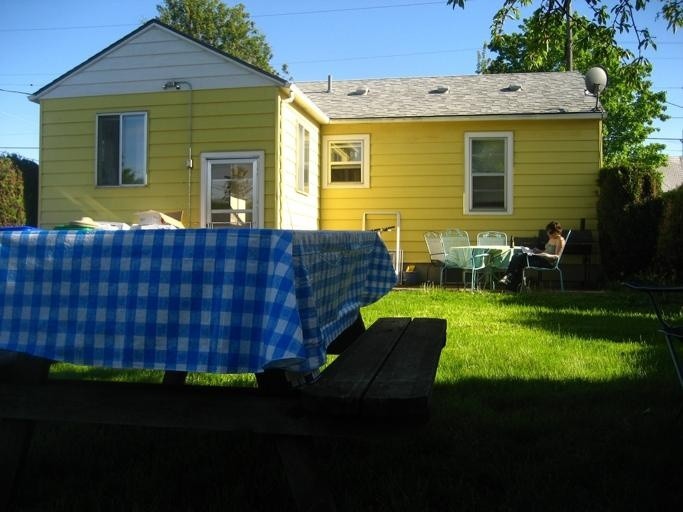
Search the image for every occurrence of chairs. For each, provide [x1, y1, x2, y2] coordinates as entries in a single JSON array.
[[420, 226, 574, 292], [617, 279, 682, 393]]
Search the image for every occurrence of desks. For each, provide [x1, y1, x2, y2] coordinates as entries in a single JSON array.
[[0, 221, 457, 512]]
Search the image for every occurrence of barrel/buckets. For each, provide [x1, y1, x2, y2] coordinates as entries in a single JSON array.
[[402, 271, 421, 287]]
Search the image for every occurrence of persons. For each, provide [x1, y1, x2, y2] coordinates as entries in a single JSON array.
[[499, 222, 566, 292]]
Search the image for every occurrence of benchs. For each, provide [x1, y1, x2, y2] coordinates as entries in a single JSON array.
[[303, 307, 454, 430]]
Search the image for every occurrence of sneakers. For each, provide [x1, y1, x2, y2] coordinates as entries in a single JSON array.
[[499, 275, 512, 286]]
